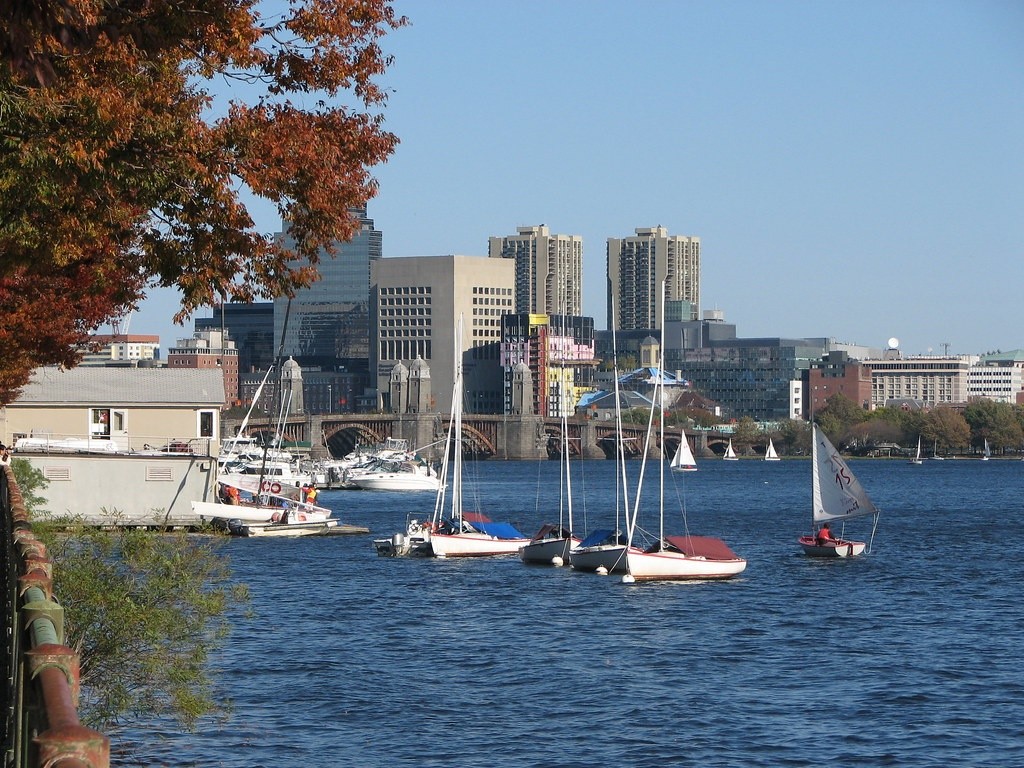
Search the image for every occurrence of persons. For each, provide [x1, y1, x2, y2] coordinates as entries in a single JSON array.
[[302, 483, 317, 510], [0, 445, 11, 467], [817, 523, 835, 544]]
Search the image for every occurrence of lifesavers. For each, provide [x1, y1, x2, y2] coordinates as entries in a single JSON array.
[[271, 483, 281, 493], [262, 481, 271, 491]]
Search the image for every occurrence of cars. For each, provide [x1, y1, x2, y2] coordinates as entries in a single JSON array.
[[845, 444, 855, 452]]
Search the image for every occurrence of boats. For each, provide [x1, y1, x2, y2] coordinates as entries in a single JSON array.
[[218, 433, 449, 501], [242, 518, 339, 538], [929, 456, 944, 460]]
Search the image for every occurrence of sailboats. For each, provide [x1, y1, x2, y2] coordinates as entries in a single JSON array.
[[625, 278, 746, 580], [979, 438, 991, 460], [517, 299, 586, 564], [374, 312, 532, 556], [722, 438, 739, 461], [569, 293, 644, 572], [670, 428, 698, 472], [798, 422, 880, 556], [190, 286, 332, 521], [764, 438, 780, 461], [907, 436, 923, 464]]
